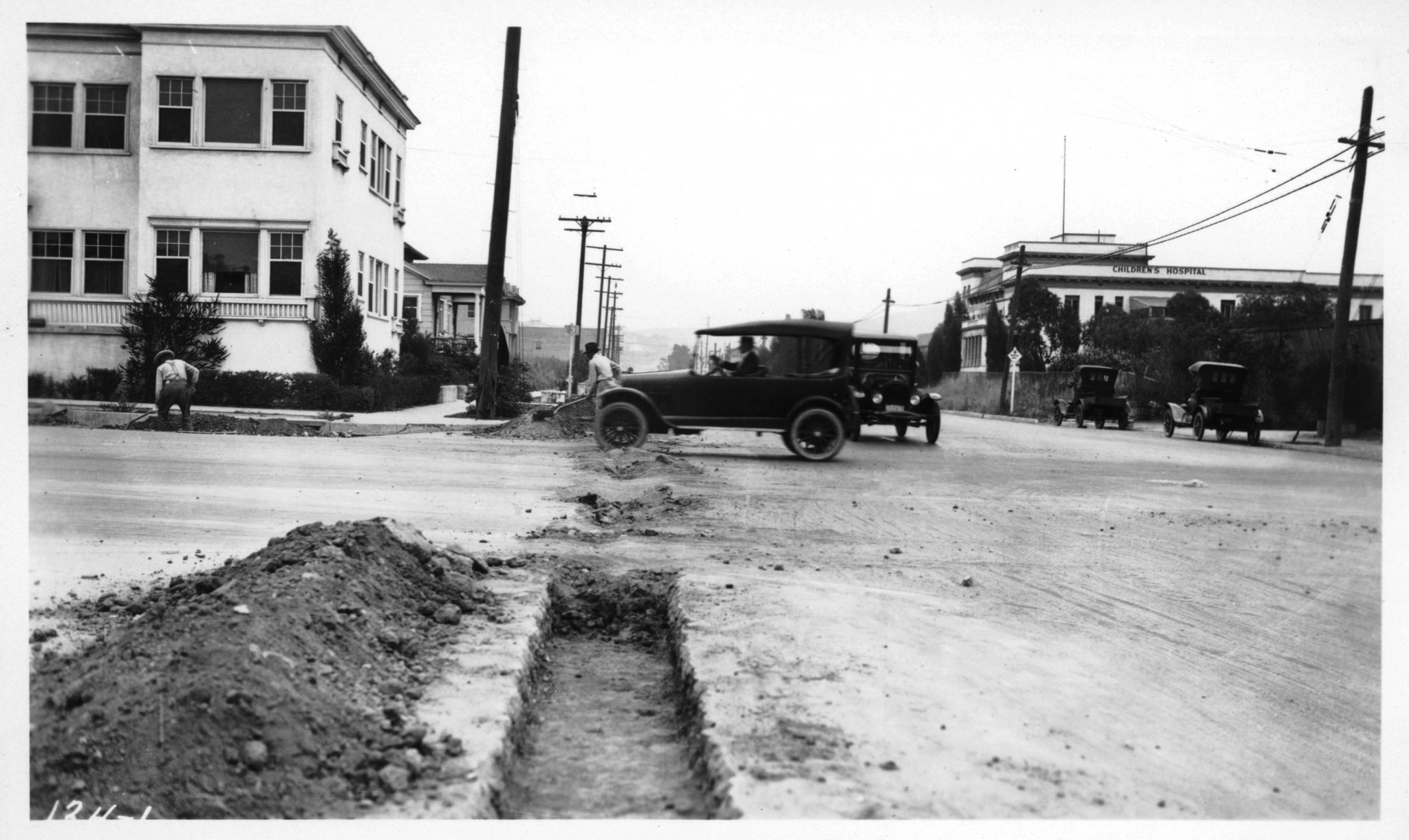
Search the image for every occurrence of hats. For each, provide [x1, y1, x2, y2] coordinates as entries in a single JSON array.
[[153, 349, 175, 366], [739, 336, 755, 345], [583, 342, 602, 355]]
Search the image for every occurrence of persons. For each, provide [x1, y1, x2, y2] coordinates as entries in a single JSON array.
[[709, 336, 758, 377], [153, 349, 199, 431], [582, 343, 620, 416]]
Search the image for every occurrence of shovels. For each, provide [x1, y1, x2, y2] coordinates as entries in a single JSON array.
[[536, 393, 596, 418]]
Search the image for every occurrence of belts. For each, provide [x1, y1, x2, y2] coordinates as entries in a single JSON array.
[[597, 378, 613, 383], [165, 378, 186, 384]]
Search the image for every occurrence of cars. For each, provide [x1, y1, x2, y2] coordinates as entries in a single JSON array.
[[1052, 365, 1128, 431], [594, 315, 866, 460], [848, 332, 941, 444], [1164, 362, 1259, 439]]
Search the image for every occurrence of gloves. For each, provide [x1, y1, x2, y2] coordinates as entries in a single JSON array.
[[153, 405, 159, 412]]
